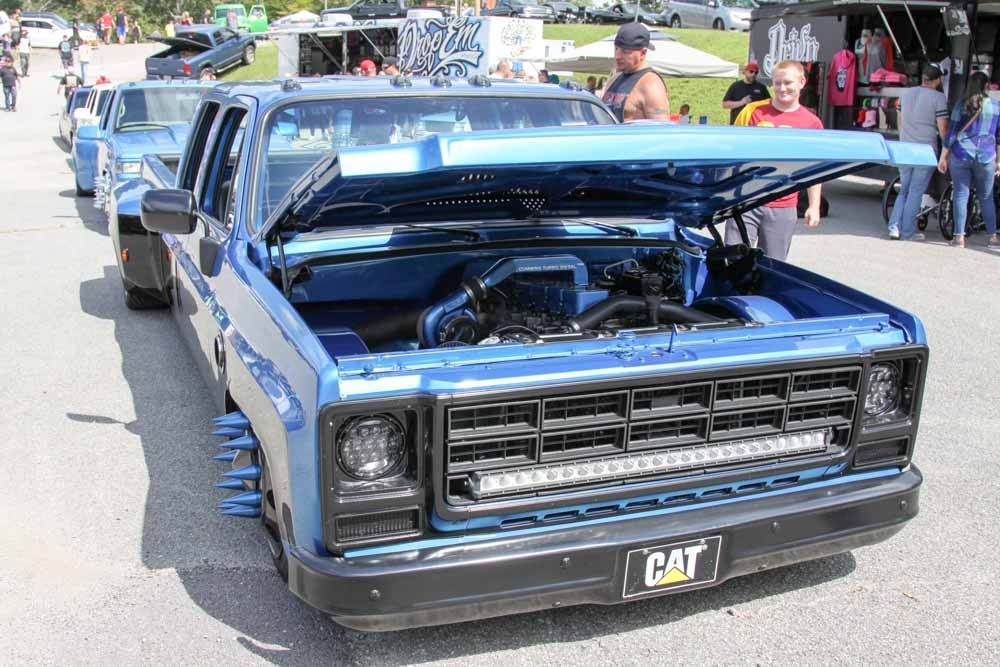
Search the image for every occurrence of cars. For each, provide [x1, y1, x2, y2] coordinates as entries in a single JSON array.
[[542, 0, 760, 33], [0, 10, 97, 49], [58, 80, 938, 631]]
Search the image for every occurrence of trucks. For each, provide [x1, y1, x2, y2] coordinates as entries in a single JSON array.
[[266, 10, 548, 119], [747, 0, 1000, 129]]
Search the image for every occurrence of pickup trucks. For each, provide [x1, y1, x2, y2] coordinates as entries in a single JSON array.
[[320, 0, 451, 21], [480, 0, 554, 24], [145, 26, 257, 81]]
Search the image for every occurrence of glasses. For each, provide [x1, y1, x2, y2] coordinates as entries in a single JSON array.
[[749, 70, 758, 75]]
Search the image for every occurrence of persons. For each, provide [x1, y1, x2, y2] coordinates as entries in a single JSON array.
[[284, 57, 607, 149], [938, 72, 1000, 248], [95, 6, 264, 45], [96, 75, 112, 85], [855, 28, 893, 87], [724, 60, 823, 262], [461, 2, 489, 17], [600, 22, 669, 122], [720, 63, 772, 125], [888, 64, 949, 242], [678, 104, 690, 124], [58, 14, 84, 99], [0, 8, 31, 112], [200, 70, 216, 81]]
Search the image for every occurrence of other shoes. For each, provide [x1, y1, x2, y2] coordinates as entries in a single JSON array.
[[949, 238, 965, 248], [13, 107, 17, 112], [909, 231, 925, 240], [6, 108, 10, 112], [988, 235, 1000, 245], [887, 224, 900, 238]]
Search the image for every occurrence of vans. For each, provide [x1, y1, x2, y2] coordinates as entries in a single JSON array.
[[210, 4, 267, 32]]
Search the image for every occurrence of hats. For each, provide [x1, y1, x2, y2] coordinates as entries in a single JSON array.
[[746, 64, 758, 70], [360, 60, 375, 71], [615, 22, 655, 51], [117, 7, 123, 11], [68, 66, 75, 73], [923, 62, 948, 80], [383, 56, 398, 64]]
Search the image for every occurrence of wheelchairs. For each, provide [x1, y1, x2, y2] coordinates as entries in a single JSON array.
[[882, 134, 986, 239]]
